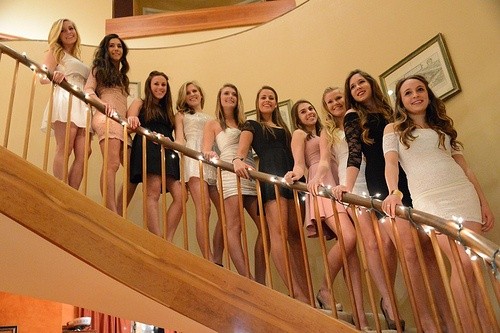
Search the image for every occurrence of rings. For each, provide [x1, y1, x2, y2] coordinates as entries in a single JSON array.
[[238, 174, 242, 176], [386, 204, 390, 207], [243, 169, 247, 171]]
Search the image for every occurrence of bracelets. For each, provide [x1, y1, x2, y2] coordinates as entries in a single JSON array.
[[390, 190, 404, 200], [232, 156, 243, 162]]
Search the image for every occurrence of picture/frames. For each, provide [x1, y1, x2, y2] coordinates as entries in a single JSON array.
[[0, 325, 18, 333], [378, 33, 462, 112], [126, 82, 141, 109], [244, 98, 294, 158]]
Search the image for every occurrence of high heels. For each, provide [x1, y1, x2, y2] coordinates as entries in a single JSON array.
[[316, 290, 343, 311], [380, 297, 405, 332]]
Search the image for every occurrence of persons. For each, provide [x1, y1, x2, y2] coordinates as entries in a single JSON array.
[[203, 83, 270, 286], [231, 85, 312, 306], [38, 18, 96, 191], [84, 33, 139, 217], [331, 69, 457, 333], [127, 71, 188, 243], [381, 75, 496, 333], [306, 87, 407, 330], [173, 82, 225, 268], [283, 100, 374, 333]]
